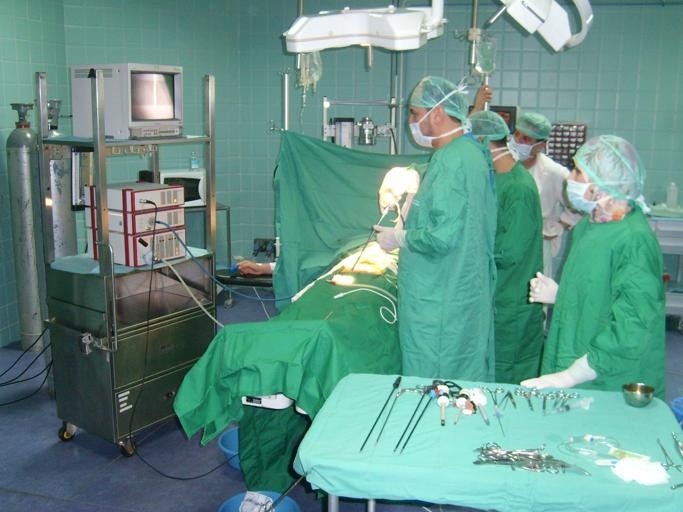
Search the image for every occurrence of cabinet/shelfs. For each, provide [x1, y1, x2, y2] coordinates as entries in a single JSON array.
[[646, 214, 683, 333]]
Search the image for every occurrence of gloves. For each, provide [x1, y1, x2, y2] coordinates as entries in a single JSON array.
[[520, 354, 596, 389], [529, 272, 558, 304], [373, 225, 407, 252]]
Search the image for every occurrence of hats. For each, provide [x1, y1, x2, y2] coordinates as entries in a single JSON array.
[[516, 113, 551, 140], [573, 135, 644, 199], [410, 75, 467, 120], [470, 110, 510, 140]]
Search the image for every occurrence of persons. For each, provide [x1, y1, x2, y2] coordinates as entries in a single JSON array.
[[374, 75, 496, 381], [465, 111, 543, 386], [468, 82, 581, 328], [520, 135, 666, 403]]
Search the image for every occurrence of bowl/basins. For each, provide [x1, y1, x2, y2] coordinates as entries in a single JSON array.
[[672, 397, 683, 425], [622, 383, 655, 408]]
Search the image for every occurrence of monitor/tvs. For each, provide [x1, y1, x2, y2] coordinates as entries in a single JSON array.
[[70, 61, 183, 140]]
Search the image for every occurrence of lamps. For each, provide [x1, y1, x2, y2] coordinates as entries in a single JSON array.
[[283, 1, 447, 68], [487, 0, 595, 53]]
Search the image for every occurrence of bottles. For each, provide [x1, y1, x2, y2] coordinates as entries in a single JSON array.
[[190, 152, 200, 172], [667, 182, 678, 208]]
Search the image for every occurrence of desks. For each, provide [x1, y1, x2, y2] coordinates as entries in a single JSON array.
[[293, 373, 683, 512], [182, 201, 231, 268]]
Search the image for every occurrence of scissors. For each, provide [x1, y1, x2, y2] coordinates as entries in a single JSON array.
[[524, 392, 536, 411], [561, 391, 580, 407], [473, 442, 572, 474], [482, 387, 505, 405], [657, 438, 683, 473], [549, 391, 567, 408], [672, 431, 683, 457], [515, 388, 525, 395]]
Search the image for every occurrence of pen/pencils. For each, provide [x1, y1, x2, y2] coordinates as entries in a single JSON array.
[[536, 392, 554, 409]]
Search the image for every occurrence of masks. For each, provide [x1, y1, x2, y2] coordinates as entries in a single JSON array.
[[409, 121, 435, 147], [508, 136, 533, 162], [566, 180, 599, 215]]
